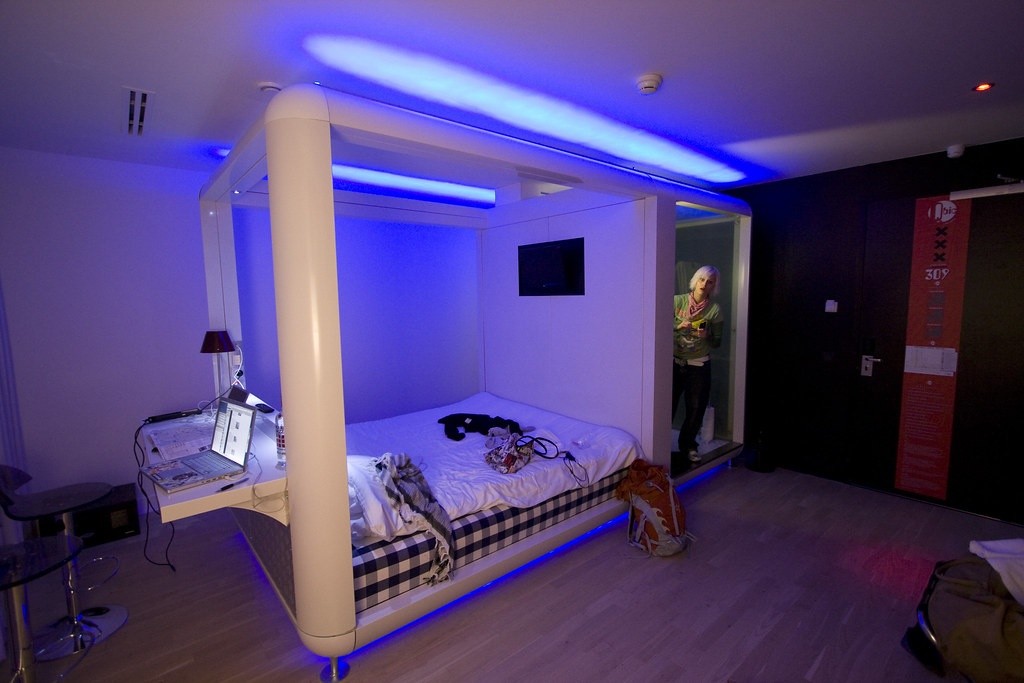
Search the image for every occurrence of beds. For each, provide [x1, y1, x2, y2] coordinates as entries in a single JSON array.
[[226, 390, 649, 683]]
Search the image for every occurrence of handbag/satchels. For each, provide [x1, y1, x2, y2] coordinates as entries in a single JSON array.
[[927, 556, 1023, 683], [483, 432, 559, 474]]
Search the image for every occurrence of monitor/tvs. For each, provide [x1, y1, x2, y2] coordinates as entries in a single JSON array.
[[518, 237, 585, 296]]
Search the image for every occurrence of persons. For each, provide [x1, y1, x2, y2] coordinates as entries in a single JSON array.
[[673, 266, 723, 462]]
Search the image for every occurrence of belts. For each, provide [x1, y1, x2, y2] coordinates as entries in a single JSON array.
[[673, 356, 709, 366]]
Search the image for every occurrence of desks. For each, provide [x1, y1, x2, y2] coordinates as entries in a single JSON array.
[[142, 412, 286, 524], [0, 533, 84, 683], [11, 481, 129, 664]]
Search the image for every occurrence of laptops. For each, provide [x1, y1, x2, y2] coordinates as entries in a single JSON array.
[[138, 396, 256, 494]]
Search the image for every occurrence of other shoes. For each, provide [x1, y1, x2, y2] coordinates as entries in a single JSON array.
[[686, 449, 702, 462]]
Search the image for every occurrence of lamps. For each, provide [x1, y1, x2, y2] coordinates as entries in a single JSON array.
[[199, 329, 247, 391]]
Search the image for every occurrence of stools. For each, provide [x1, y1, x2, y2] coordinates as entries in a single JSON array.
[[0, 465, 43, 537]]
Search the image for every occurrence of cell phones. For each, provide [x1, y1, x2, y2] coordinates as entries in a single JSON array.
[[699, 321, 706, 329], [256, 404, 275, 413]]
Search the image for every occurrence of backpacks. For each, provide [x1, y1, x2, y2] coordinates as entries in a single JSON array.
[[615, 458, 687, 556]]
